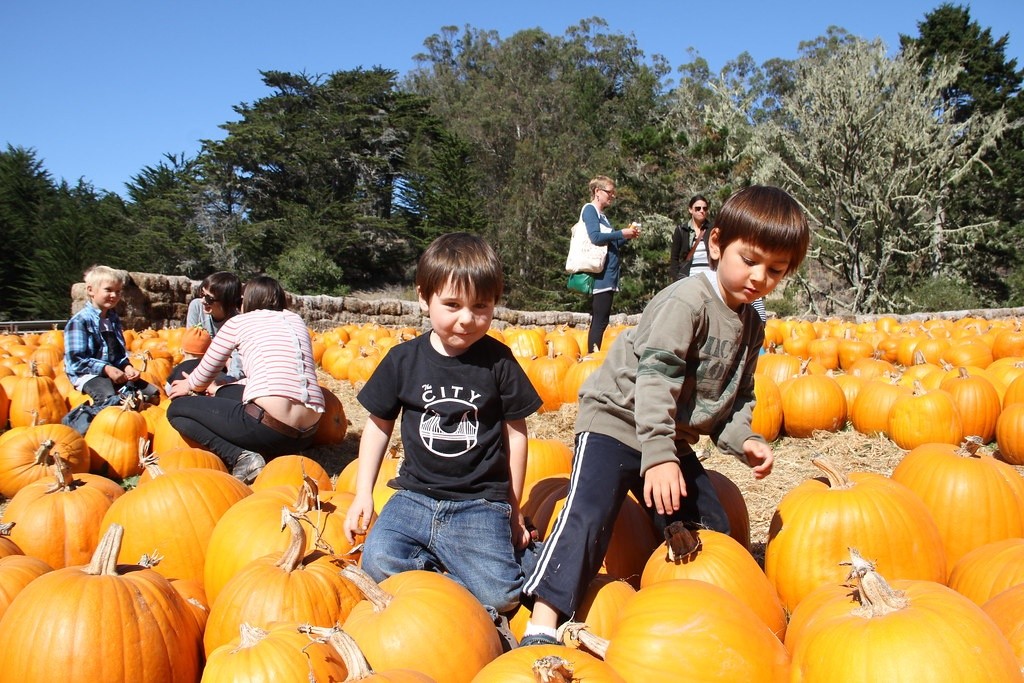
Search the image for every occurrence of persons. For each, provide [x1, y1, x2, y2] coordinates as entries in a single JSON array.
[[63, 264, 161, 409], [164, 322, 238, 396], [185, 269, 245, 381], [669, 195, 718, 281], [166, 276, 327, 482], [515, 187, 812, 647], [751, 296, 767, 355], [344, 227, 545, 615], [580, 172, 640, 355]]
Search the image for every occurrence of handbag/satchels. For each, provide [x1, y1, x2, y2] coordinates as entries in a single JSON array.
[[567, 271, 595, 295], [565, 203, 612, 273]]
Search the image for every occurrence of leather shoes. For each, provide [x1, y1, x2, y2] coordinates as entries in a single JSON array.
[[231, 450, 266, 484]]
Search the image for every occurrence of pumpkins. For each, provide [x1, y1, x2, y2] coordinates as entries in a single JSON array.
[[0, 312, 1024, 683]]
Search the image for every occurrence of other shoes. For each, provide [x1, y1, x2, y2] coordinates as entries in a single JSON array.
[[519, 631, 559, 648]]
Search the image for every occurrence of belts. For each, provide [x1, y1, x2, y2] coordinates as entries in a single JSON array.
[[244, 402, 319, 439]]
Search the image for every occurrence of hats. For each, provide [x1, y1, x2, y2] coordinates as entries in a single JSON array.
[[182, 323, 211, 354]]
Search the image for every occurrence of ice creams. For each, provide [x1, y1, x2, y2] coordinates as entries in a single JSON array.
[[630, 222, 638, 229]]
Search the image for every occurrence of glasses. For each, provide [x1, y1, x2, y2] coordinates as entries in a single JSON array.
[[199, 291, 220, 305], [695, 207, 708, 212], [594, 187, 616, 198]]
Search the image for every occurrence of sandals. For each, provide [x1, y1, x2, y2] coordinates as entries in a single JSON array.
[[490, 612, 519, 653]]
